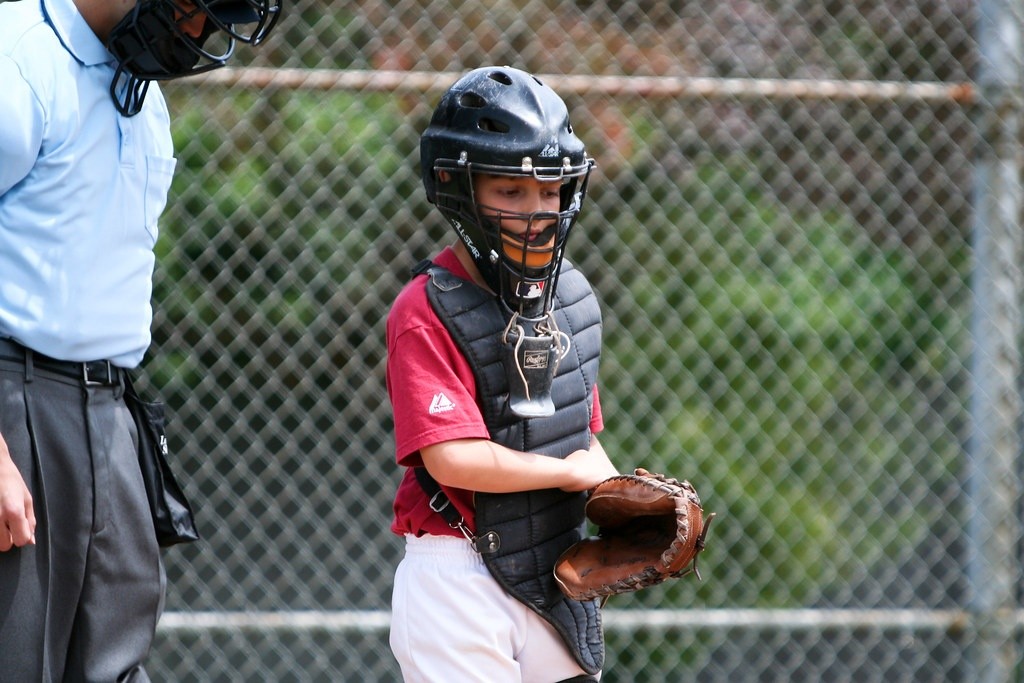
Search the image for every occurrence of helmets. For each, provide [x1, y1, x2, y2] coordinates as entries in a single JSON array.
[[420, 65, 597, 322], [108, 0, 283, 118]]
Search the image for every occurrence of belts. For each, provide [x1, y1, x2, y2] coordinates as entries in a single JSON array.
[[0, 337, 127, 386]]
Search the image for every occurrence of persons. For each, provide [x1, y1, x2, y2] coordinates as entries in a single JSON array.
[[385, 64, 716, 683], [0, 0, 291, 683]]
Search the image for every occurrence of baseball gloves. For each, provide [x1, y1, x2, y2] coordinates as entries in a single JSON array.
[[550, 466, 719, 610]]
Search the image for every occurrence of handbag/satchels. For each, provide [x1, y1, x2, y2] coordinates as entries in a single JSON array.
[[122, 373, 200, 547]]
